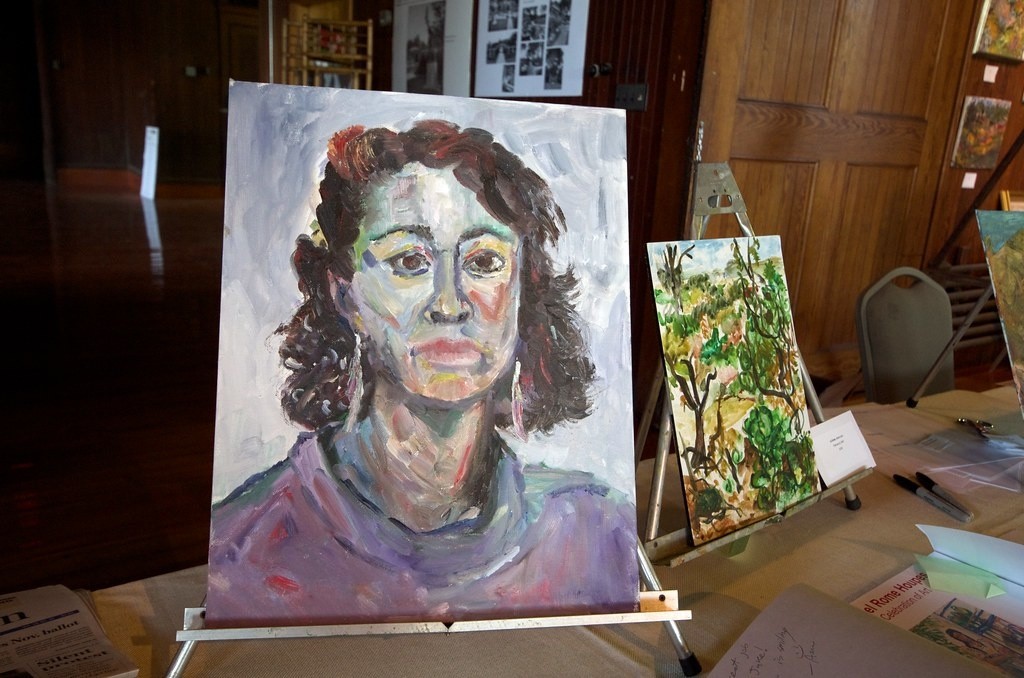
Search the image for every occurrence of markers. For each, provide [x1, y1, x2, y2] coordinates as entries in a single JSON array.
[[915, 471, 975, 521], [893, 474, 970, 524]]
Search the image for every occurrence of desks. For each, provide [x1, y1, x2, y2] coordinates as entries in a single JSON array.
[[0, 371, 1024, 678]]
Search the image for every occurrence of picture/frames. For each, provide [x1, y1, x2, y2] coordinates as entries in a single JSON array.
[[468, 0, 592, 99]]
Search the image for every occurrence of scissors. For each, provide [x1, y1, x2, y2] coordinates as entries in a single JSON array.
[[956, 417, 995, 442]]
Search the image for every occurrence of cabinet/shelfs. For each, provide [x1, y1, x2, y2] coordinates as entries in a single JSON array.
[[280, 12, 375, 90]]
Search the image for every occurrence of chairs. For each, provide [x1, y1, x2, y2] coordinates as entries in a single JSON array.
[[854, 266, 958, 406]]
